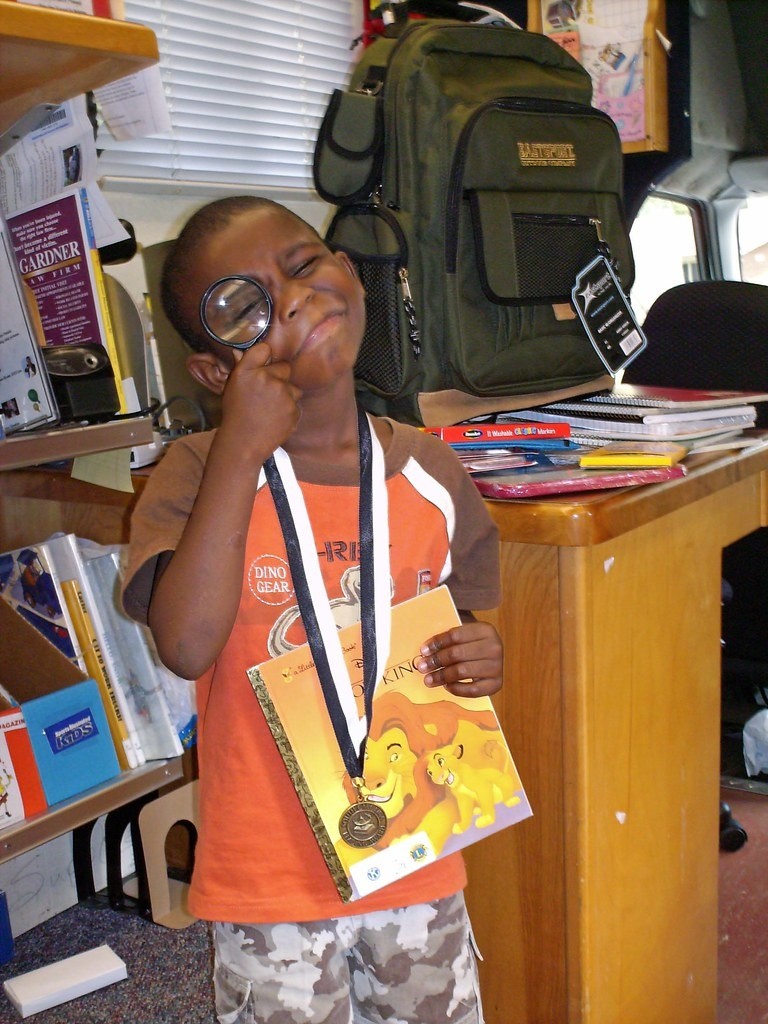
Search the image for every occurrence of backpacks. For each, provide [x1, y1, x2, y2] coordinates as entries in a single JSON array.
[[310, 0, 636, 430]]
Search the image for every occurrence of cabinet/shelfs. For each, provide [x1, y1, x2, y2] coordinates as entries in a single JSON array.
[[0, 0, 202, 922]]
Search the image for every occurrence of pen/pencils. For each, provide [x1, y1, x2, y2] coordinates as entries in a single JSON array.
[[464, 454, 538, 470]]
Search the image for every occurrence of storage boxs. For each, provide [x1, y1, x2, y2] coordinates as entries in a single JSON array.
[[0, 709, 49, 833], [21, 679, 130, 807]]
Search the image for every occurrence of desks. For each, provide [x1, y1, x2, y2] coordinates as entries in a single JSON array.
[[457, 422, 768, 1024]]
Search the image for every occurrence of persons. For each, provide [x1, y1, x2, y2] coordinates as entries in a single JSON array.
[[120, 195, 505, 1024]]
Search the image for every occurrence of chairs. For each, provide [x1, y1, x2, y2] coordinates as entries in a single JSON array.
[[614, 279, 768, 855]]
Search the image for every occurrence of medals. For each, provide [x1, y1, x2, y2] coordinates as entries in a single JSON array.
[[338, 802, 388, 849]]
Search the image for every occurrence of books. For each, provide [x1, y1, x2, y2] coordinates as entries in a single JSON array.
[[0, 531, 197, 771], [416, 383, 768, 500], [245, 584, 533, 904], [0, 186, 127, 433]]
